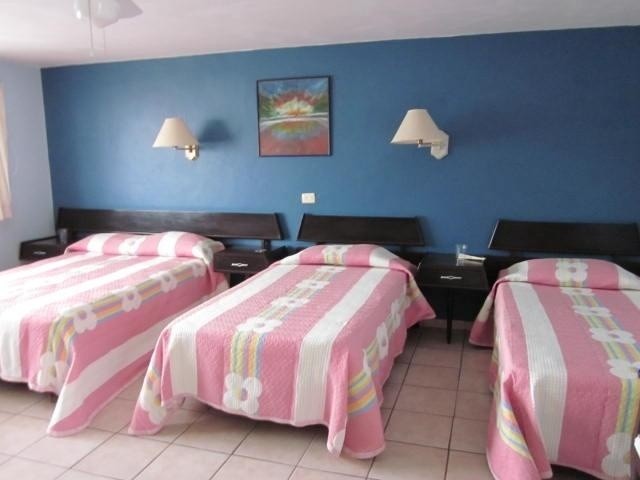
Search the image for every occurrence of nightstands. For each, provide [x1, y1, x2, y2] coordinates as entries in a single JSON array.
[[214, 249, 286, 280], [415, 253, 490, 343], [20, 236, 82, 260]]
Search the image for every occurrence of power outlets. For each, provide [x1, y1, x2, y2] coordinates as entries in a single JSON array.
[[301, 192, 315, 204]]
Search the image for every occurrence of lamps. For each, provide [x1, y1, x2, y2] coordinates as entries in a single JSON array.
[[153, 116, 199, 160], [391, 109, 449, 160], [73, 0, 141, 58]]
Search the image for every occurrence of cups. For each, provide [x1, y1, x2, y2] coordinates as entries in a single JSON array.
[[456, 245, 466, 259]]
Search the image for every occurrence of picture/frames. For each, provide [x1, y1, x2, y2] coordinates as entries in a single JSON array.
[[256, 76, 331, 157]]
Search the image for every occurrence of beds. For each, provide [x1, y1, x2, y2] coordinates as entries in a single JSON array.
[[0, 208, 283, 441], [128, 212, 427, 460], [469, 218, 640, 480]]
